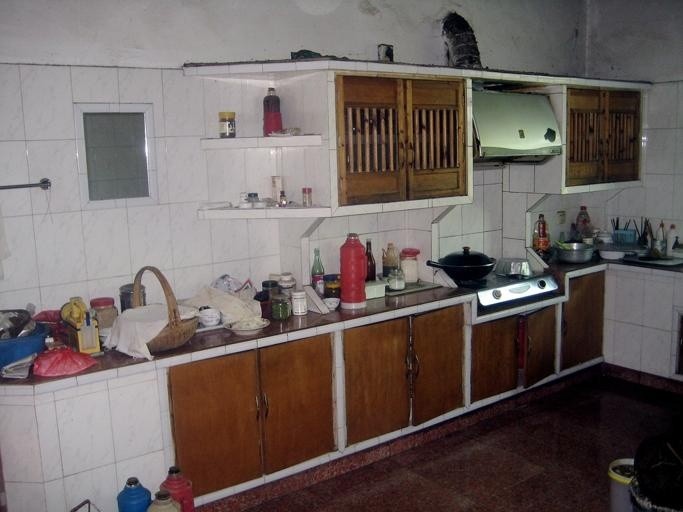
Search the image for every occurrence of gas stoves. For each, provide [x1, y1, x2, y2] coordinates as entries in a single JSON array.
[[446, 270, 560, 312]]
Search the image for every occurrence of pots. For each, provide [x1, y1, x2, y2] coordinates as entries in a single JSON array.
[[424, 247, 497, 280]]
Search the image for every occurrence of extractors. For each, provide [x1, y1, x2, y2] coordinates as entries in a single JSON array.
[[442, 13, 562, 165]]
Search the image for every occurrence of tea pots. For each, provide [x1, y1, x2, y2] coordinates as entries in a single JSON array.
[[381, 243, 399, 276]]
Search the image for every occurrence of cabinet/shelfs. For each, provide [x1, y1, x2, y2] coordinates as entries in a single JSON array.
[[342, 301, 464, 448], [568, 89, 639, 185], [197, 132, 333, 219], [334, 74, 465, 202], [469, 305, 557, 406], [560, 269, 606, 370], [167, 328, 333, 498]]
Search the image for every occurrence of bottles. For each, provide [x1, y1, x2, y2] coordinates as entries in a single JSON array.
[[533, 213, 551, 261], [644, 225, 651, 250], [388, 269, 405, 290], [655, 224, 665, 241], [279, 192, 286, 207], [559, 232, 565, 242], [577, 204, 590, 229], [248, 193, 258, 201], [364, 239, 375, 282], [667, 224, 678, 258], [272, 175, 282, 202], [119, 284, 146, 311], [312, 248, 325, 296], [302, 188, 312, 206], [263, 87, 282, 135], [279, 272, 296, 309]]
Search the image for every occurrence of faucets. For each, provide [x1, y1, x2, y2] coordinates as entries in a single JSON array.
[[672, 236, 683, 250]]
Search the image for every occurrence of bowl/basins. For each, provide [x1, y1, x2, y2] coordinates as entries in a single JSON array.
[[324, 297, 341, 311], [98, 327, 117, 344], [613, 229, 637, 246], [495, 256, 533, 277], [221, 317, 269, 336], [597, 249, 626, 260], [552, 243, 595, 264], [198, 310, 221, 327]]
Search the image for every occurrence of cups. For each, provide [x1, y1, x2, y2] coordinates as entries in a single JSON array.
[[582, 238, 593, 245], [652, 239, 666, 256], [590, 228, 600, 237]]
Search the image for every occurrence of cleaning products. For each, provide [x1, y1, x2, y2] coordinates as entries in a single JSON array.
[[666, 224, 679, 257]]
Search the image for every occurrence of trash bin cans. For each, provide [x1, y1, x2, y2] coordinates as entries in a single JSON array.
[[630, 433, 683, 512]]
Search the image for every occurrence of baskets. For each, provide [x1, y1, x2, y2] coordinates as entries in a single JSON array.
[[133, 266, 198, 354]]
